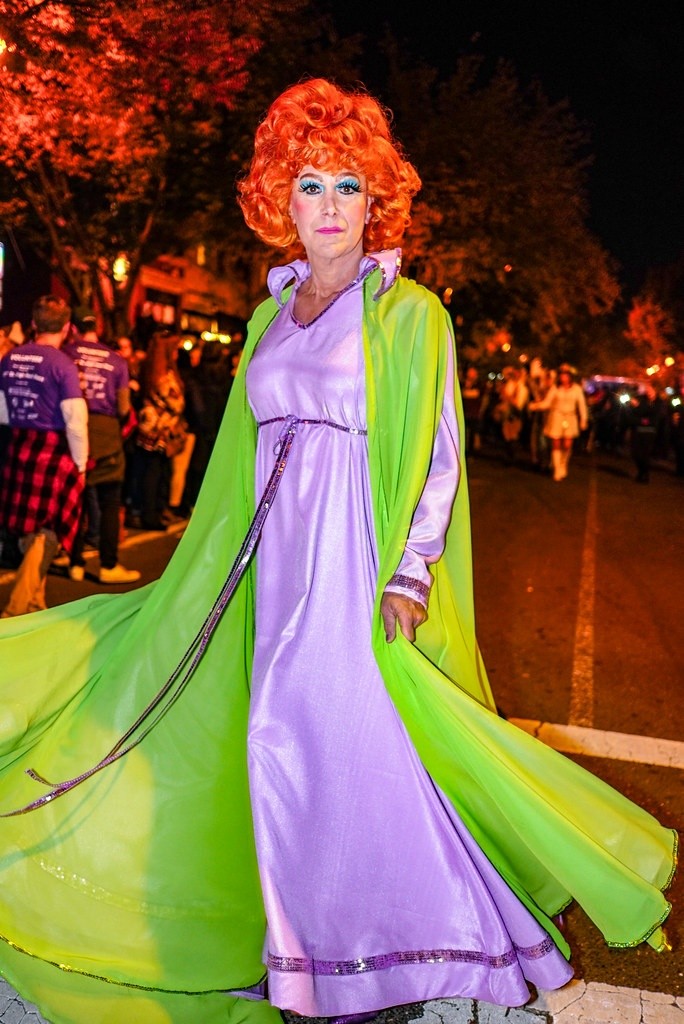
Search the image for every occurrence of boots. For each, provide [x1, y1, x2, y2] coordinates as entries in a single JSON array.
[[552, 448, 570, 481]]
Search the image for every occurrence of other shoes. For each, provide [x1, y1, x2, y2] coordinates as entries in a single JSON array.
[[123, 516, 168, 532]]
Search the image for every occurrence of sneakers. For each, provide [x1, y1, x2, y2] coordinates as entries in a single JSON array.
[[100, 564, 141, 581], [71, 566, 83, 581]]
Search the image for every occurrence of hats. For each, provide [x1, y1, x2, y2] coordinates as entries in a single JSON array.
[[71, 307, 96, 324]]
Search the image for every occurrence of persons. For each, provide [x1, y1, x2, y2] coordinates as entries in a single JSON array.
[[1, 78, 678, 1024], [462, 356, 684, 483], [0, 295, 248, 618]]
